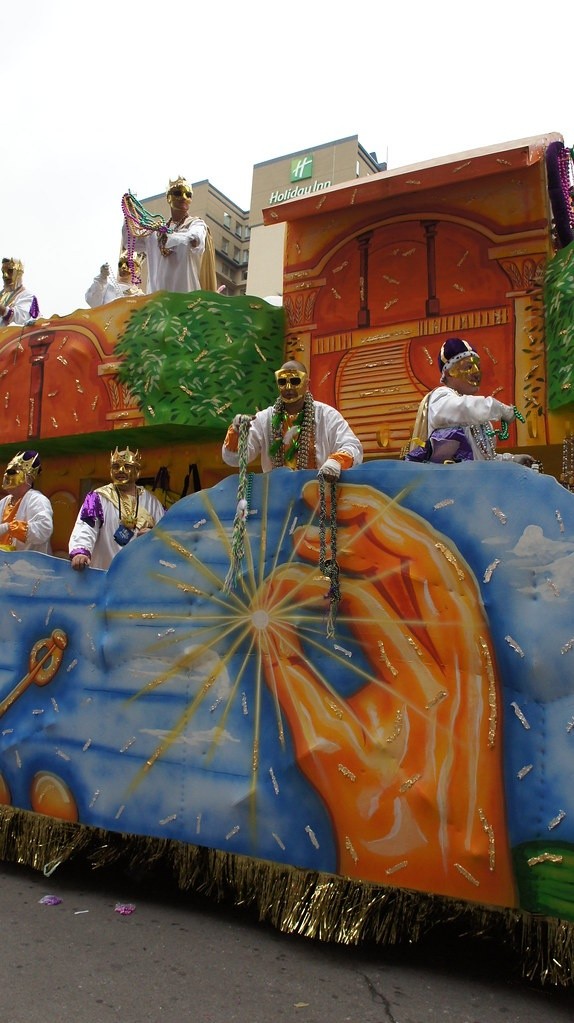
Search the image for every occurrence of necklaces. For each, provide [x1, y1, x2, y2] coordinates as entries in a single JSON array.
[[116, 485, 138, 517], [268, 390, 317, 469], [458, 393, 525, 460], [122, 193, 190, 284], [10, 495, 21, 506], [107, 264, 124, 297]]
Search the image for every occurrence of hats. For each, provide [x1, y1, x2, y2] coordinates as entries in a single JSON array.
[[11, 451, 42, 480], [438, 338, 481, 375]]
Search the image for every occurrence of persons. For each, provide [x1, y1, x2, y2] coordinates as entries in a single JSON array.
[[222, 360, 364, 484], [0, 257, 40, 326], [410, 337, 537, 468], [0, 451, 53, 554], [68, 447, 165, 570], [85, 175, 218, 307]]
